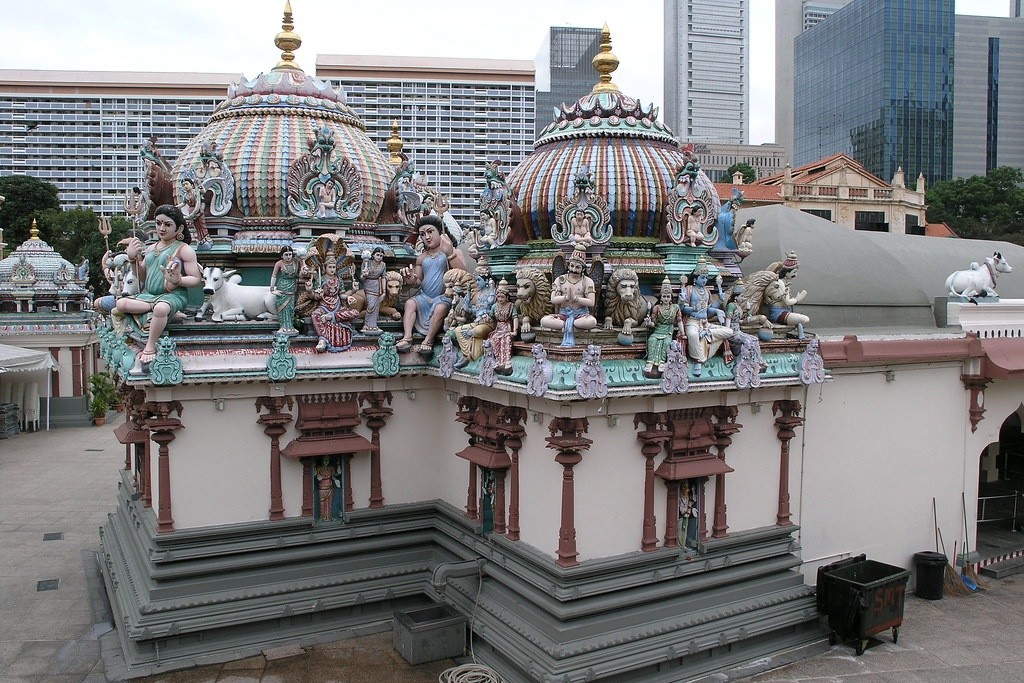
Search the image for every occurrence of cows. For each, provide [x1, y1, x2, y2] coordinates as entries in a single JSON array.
[[194, 262, 277, 322], [944, 251, 1013, 305], [121, 268, 144, 297]]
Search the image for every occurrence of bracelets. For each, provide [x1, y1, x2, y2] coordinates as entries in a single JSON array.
[[795, 298, 799, 304], [574, 296, 579, 303], [447, 253, 456, 261], [564, 295, 569, 302]]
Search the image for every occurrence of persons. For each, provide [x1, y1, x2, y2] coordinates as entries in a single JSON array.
[[270, 246, 306, 334], [313, 456, 342, 520], [305, 256, 359, 351], [441, 265, 517, 376], [768, 258, 810, 326], [642, 264, 759, 373], [463, 210, 498, 249], [394, 214, 466, 354], [741, 218, 755, 248], [359, 247, 387, 331], [318, 178, 336, 218], [541, 252, 598, 331], [117, 204, 201, 363], [679, 479, 698, 547], [687, 205, 704, 247], [95, 229, 147, 312], [482, 471, 495, 523], [174, 179, 213, 249], [569, 209, 592, 243]]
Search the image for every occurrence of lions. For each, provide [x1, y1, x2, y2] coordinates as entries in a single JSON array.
[[603, 269, 648, 334], [348, 270, 404, 320], [515, 267, 553, 333], [443, 268, 479, 322], [739, 270, 783, 328]]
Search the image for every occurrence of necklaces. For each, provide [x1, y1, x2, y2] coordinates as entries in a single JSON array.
[[567, 274, 582, 285], [576, 220, 583, 227], [282, 261, 295, 275], [429, 250, 441, 259], [154, 240, 178, 257], [659, 305, 673, 319]]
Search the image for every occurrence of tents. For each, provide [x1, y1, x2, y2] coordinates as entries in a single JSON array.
[[0, 343, 60, 431]]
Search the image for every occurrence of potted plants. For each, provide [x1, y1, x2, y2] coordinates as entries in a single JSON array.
[[109, 393, 124, 413], [88, 374, 116, 426]]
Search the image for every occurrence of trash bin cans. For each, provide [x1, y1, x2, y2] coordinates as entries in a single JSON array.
[[913, 551, 949, 601], [815, 553, 912, 656]]
